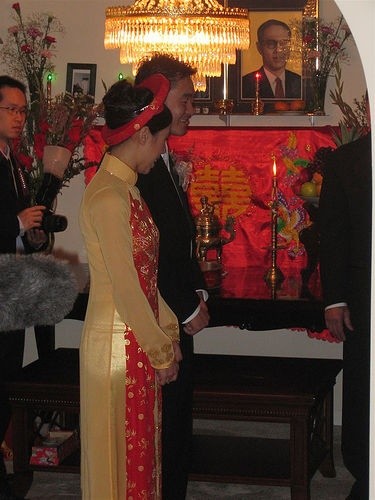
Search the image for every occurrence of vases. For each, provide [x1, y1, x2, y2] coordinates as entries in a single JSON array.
[[304, 73, 328, 115]]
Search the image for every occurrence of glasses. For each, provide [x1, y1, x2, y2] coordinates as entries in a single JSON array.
[[260, 40, 291, 49], [0, 106, 31, 115]]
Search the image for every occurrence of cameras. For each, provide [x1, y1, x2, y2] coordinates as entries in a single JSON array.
[[33, 210, 68, 236]]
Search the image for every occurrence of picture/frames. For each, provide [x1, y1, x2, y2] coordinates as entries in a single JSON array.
[[236, 7, 307, 104], [65, 62, 97, 105], [191, 76, 215, 104]]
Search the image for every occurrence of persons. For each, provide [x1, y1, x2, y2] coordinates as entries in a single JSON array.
[[318, 91, 371, 500], [0, 74, 48, 458], [242, 18, 301, 99], [78, 71, 182, 500], [132, 53, 211, 500]]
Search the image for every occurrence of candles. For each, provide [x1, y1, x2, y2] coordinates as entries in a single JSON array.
[[272, 154, 279, 188]]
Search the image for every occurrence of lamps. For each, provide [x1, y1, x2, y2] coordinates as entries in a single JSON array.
[[103, 0, 250, 94]]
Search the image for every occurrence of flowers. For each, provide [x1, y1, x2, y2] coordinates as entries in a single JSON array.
[[7, 2, 85, 170], [302, 13, 351, 76]]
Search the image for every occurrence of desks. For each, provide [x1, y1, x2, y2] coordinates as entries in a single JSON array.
[[0, 347, 344, 500]]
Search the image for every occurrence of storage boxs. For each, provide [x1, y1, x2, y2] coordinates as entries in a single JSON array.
[[31, 430, 79, 466]]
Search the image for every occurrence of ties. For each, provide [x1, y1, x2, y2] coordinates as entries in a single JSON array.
[[275, 78, 284, 97]]
